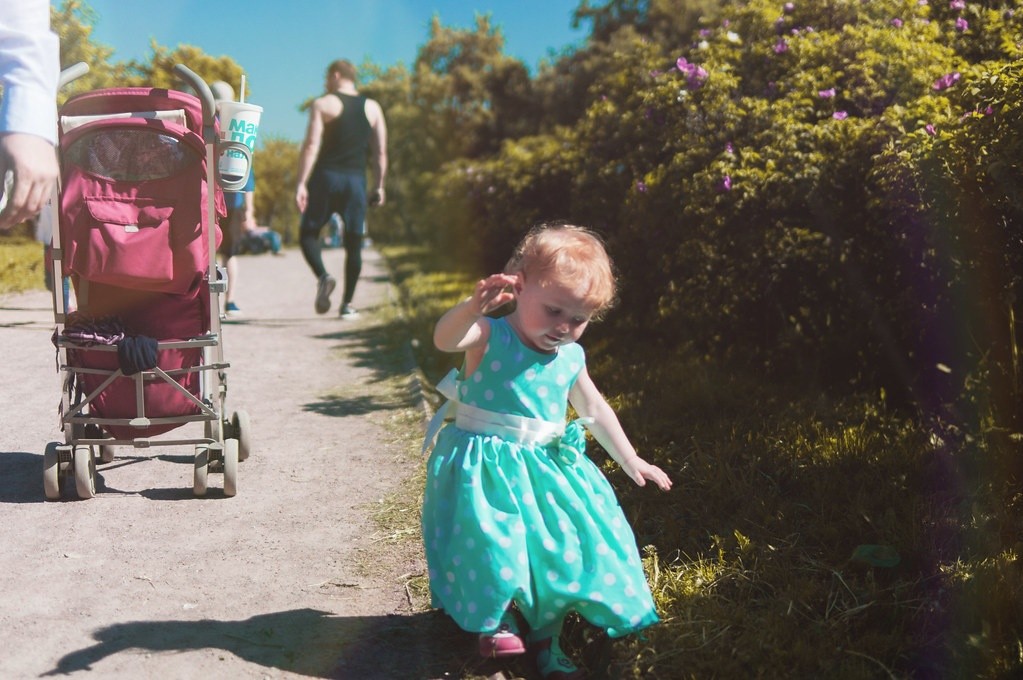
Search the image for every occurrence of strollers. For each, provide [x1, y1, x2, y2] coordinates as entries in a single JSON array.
[[42, 62, 250, 500]]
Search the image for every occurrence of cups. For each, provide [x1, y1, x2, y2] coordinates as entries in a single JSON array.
[[219, 101, 263, 177]]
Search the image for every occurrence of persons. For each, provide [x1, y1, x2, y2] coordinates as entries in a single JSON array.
[[327, 213, 340, 247], [36, 205, 76, 313], [211, 80, 254, 315], [295, 58, 387, 321], [248, 217, 281, 256], [423, 225, 673, 680], [0, 0, 60, 231]]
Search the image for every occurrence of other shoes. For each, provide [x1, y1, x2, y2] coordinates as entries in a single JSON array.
[[479, 602, 526, 658], [315, 277, 336, 314], [520, 624, 584, 680], [340, 306, 359, 319], [225, 309, 250, 318]]
